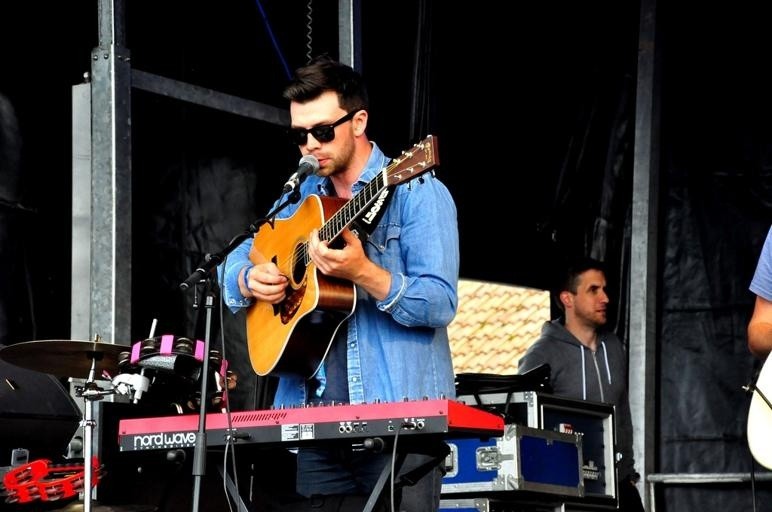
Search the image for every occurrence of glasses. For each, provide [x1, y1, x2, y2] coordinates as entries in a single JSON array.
[[286, 112, 354, 146]]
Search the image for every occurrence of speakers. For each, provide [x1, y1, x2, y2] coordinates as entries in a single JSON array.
[[0, 344, 83, 467]]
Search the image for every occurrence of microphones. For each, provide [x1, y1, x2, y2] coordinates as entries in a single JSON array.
[[283, 154, 320, 194]]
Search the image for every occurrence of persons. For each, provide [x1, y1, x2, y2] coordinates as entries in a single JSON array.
[[513, 258, 647, 511], [746, 223, 771, 356], [213, 58, 465, 510]]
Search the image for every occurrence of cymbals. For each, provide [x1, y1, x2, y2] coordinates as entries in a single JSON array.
[[0, 338, 131, 382]]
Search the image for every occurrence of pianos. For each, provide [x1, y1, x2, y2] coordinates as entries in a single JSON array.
[[115, 397, 508, 453]]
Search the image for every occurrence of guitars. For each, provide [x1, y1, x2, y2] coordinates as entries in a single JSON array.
[[243, 131, 439, 380]]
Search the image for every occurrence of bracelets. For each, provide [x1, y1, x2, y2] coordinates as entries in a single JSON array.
[[243, 265, 259, 291]]
